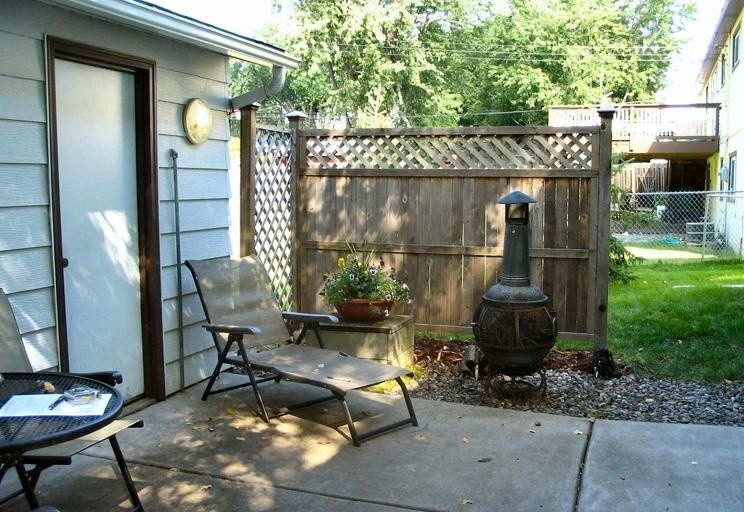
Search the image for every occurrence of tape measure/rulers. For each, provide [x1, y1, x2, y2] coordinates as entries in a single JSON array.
[[65, 385, 101, 405]]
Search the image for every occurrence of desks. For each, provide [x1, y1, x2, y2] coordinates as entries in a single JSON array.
[[0, 370, 124, 510]]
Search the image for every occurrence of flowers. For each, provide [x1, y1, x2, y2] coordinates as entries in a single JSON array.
[[318, 253, 417, 321]]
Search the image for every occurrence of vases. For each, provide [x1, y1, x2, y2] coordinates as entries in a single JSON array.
[[331, 297, 394, 324]]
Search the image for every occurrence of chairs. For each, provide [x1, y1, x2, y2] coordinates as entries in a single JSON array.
[[184, 251, 421, 448], [0, 288, 147, 511]]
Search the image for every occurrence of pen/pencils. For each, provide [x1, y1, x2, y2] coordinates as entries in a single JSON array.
[[49, 396, 65, 410]]
